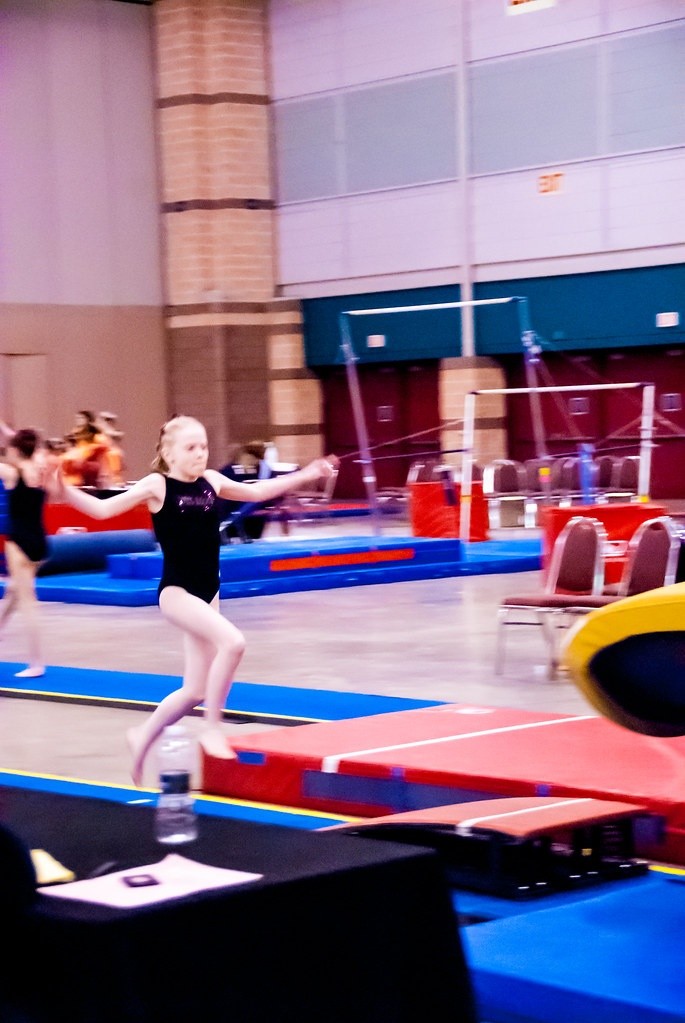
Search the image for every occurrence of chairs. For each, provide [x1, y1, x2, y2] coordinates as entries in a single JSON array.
[[534, 517, 679, 677], [490, 516, 606, 693], [409, 456, 636, 513]]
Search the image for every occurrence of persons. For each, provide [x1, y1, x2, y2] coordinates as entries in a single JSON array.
[[56, 411, 130, 489], [34, 414, 338, 788], [0, 419, 49, 680], [217, 442, 288, 547]]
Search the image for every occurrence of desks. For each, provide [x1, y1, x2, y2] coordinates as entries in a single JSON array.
[[409, 481, 491, 542], [542, 506, 668, 591], [1, 785, 479, 1023]]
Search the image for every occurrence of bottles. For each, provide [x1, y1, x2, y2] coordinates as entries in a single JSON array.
[[154, 725, 199, 843]]
